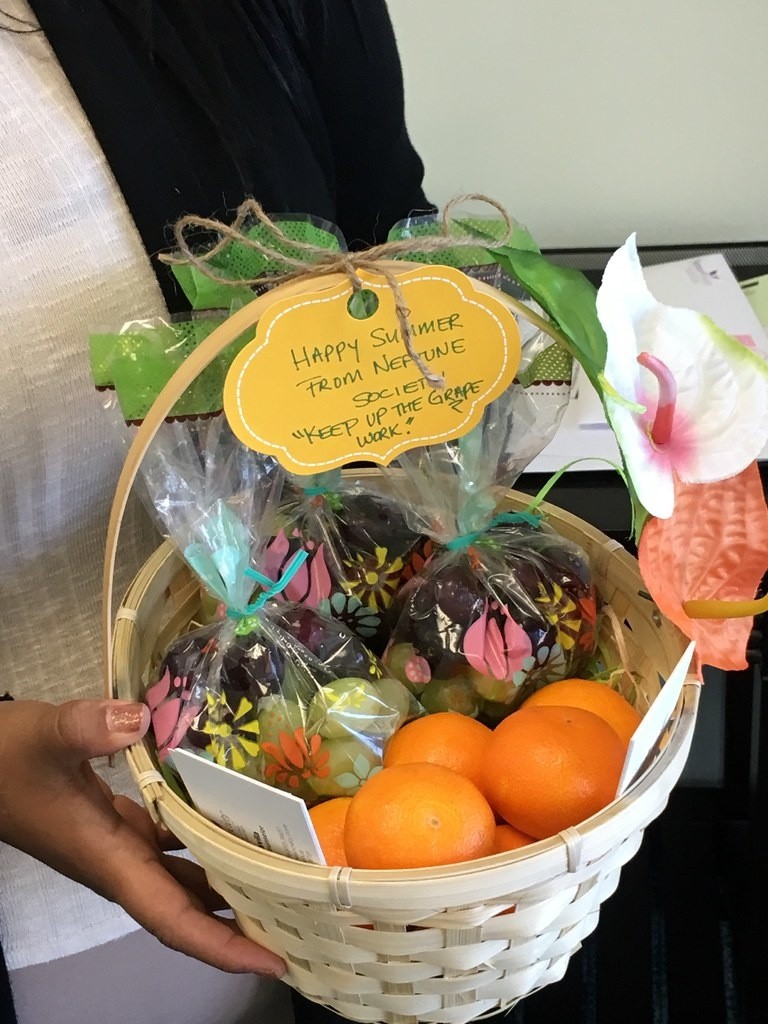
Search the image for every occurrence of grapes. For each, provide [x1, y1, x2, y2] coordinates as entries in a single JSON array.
[[165, 492, 598, 809]]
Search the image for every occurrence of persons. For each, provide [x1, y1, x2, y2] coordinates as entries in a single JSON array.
[[0, 0, 440, 1024]]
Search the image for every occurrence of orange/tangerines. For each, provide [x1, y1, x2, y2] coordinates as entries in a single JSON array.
[[301, 679, 647, 872]]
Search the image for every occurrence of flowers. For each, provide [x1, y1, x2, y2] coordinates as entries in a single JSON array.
[[596, 232, 767, 671]]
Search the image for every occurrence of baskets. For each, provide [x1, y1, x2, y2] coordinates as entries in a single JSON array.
[[103, 190, 703, 1024]]
